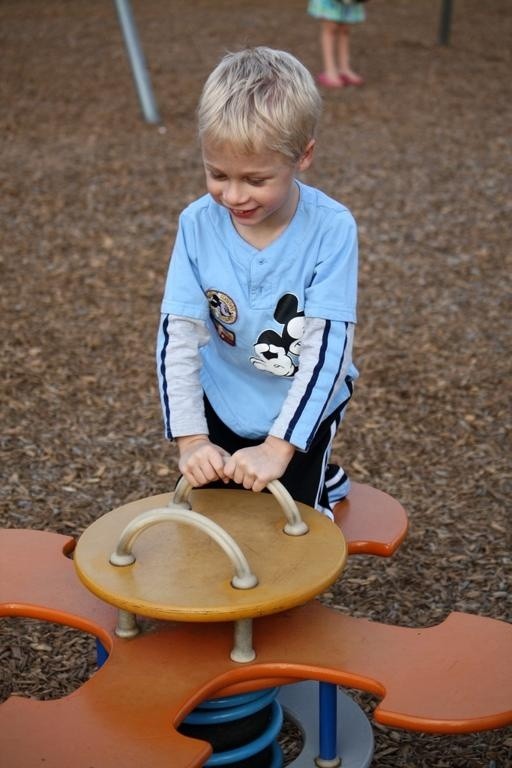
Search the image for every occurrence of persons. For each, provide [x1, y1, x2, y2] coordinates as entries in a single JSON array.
[[153, 50, 363, 527], [306, 0, 371, 89]]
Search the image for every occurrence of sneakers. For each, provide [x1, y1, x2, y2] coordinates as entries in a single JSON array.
[[319, 70, 364, 88]]
